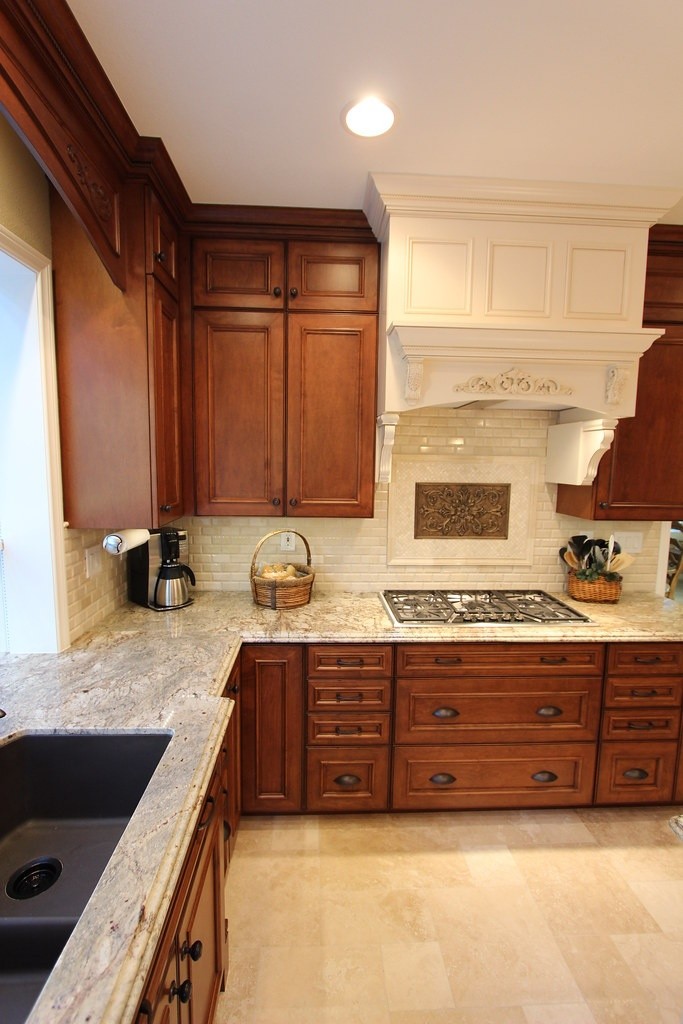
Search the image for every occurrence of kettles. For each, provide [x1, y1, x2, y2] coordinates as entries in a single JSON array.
[[154, 561, 196, 607]]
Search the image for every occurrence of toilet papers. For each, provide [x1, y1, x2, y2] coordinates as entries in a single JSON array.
[[102, 528, 151, 556]]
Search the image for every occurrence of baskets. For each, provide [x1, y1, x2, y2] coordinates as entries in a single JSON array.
[[568, 567, 623, 604], [250, 529, 315, 610]]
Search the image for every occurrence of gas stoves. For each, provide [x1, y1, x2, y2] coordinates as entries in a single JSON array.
[[378, 589, 600, 629]]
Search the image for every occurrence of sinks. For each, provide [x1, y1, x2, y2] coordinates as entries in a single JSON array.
[[0, 728, 176, 921], [0, 920, 82, 1023]]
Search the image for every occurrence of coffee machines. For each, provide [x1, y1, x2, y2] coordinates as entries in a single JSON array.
[[127, 527, 196, 611]]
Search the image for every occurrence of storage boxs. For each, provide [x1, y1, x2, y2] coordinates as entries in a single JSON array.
[[567, 568, 624, 604], [250, 530, 315, 610]]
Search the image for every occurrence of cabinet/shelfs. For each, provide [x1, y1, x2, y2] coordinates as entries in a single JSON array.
[[242, 647, 305, 813], [393, 644, 607, 810], [187, 218, 381, 520], [308, 646, 390, 812], [595, 642, 682, 808], [52, 152, 185, 531], [132, 748, 232, 1024], [555, 223, 683, 522]]
